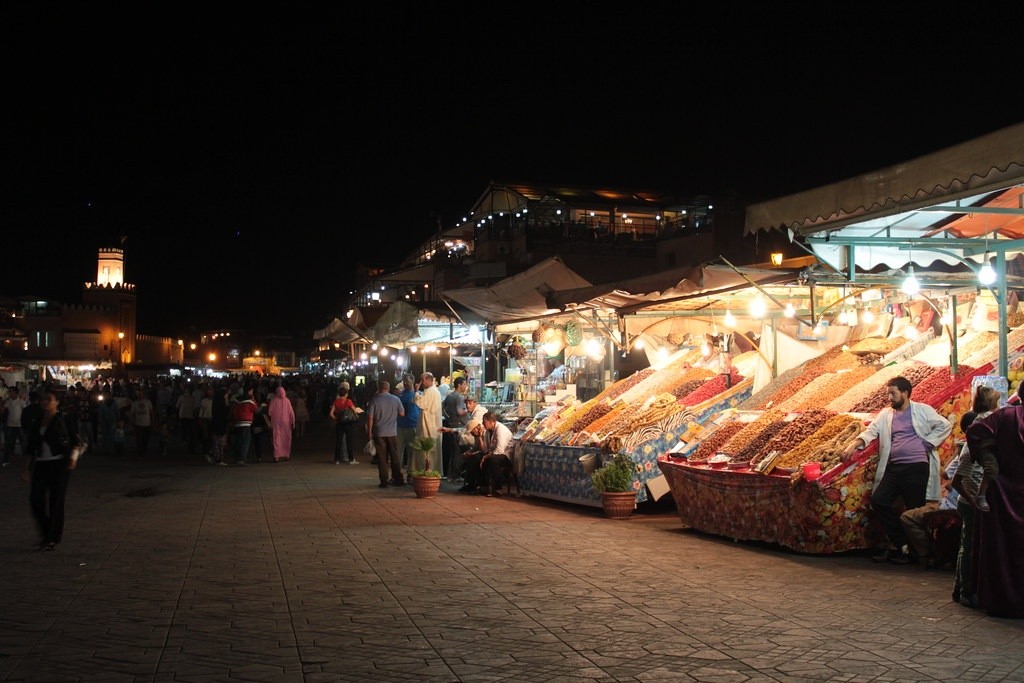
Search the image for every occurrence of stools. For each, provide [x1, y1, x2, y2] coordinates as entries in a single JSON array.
[[496, 465, 522, 495]]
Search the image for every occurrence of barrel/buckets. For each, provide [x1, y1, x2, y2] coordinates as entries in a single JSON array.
[[579, 453, 598, 472]]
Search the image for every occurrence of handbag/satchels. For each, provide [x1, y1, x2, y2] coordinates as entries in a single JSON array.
[[338, 409, 358, 422], [364, 441, 377, 458]]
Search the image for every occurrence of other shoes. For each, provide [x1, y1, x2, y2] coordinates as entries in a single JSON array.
[[274, 455, 291, 463], [204, 455, 227, 466], [873, 552, 1023, 616], [335, 460, 360, 465], [0, 460, 10, 469], [33, 539, 59, 551], [378, 480, 406, 488], [231, 457, 264, 465]]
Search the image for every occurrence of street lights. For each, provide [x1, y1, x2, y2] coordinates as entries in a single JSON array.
[[117, 329, 124, 366]]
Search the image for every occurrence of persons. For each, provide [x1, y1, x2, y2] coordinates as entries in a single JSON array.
[[409, 371, 446, 480], [897, 379, 1024, 617], [436, 396, 488, 496], [0, 335, 429, 472], [366, 378, 406, 488], [458, 419, 503, 497], [18, 391, 54, 484], [476, 411, 516, 496], [18, 385, 79, 552], [840, 375, 953, 564], [437, 376, 471, 485]]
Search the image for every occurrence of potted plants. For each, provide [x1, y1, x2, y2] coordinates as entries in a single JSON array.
[[407, 434, 444, 500], [589, 449, 641, 522]]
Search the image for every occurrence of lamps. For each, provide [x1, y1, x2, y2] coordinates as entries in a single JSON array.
[[353, 240, 1008, 369]]
